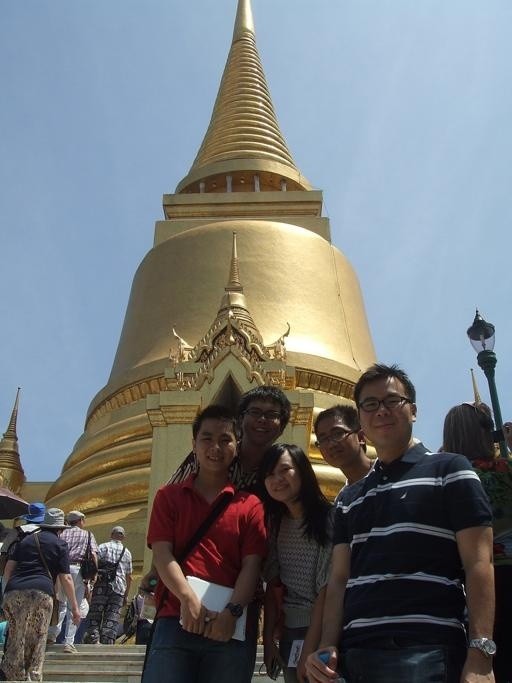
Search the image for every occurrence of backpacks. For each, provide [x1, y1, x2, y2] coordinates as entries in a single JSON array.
[[12, 526, 43, 545]]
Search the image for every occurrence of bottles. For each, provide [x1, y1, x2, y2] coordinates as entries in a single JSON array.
[[318, 653, 346, 683]]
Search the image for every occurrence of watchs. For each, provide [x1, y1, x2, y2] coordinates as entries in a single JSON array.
[[227, 604, 244, 617], [466, 637, 497, 658]]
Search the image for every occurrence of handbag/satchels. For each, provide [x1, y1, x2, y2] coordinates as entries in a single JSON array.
[[78, 530, 100, 581], [97, 543, 127, 580], [139, 493, 236, 683]]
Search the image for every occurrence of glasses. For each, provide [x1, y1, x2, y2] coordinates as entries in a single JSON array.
[[356, 392, 413, 410], [312, 428, 366, 450], [462, 400, 487, 420], [239, 406, 291, 423], [256, 464, 300, 478]]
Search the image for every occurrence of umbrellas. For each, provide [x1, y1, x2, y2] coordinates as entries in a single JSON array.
[[0, 487, 32, 517]]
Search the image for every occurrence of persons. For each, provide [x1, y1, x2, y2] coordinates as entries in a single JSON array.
[[1, 508, 79, 683], [47, 511, 101, 654], [259, 445, 334, 682], [136, 377, 293, 596], [84, 526, 133, 645], [306, 364, 497, 682], [313, 403, 378, 503], [141, 406, 269, 683], [438, 401, 511, 681], [0, 502, 46, 574]]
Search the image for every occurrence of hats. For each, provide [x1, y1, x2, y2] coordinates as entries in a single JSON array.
[[110, 526, 127, 537], [67, 510, 85, 523], [19, 502, 46, 522], [36, 507, 72, 530]]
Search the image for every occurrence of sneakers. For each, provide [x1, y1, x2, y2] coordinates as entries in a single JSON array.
[[62, 643, 78, 654]]
